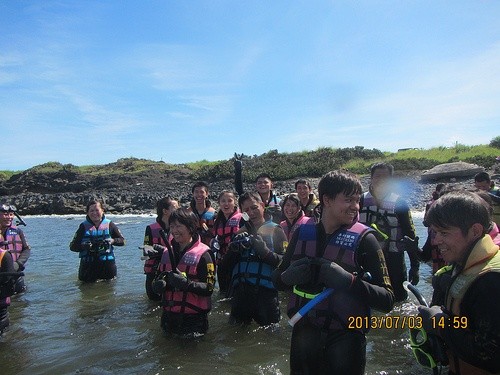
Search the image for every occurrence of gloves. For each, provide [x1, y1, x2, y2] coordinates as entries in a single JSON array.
[[316, 257, 351, 290], [81, 241, 92, 251], [250, 234, 268, 256], [13, 261, 19, 271], [103, 238, 112, 248], [234, 232, 248, 240], [416, 304, 448, 336], [166, 267, 187, 289], [399, 235, 421, 257], [139, 244, 158, 255], [281, 257, 312, 286], [153, 275, 166, 291], [408, 269, 419, 285], [153, 244, 165, 252]]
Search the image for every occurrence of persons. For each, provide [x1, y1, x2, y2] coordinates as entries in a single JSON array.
[[274, 172, 395, 375], [417, 189, 500, 375], [0, 204, 31, 336], [69, 201, 126, 282], [359, 162, 420, 301], [400, 172, 500, 277], [139, 174, 317, 336]]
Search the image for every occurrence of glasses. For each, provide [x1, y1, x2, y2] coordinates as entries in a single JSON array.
[[1, 205, 17, 211]]
